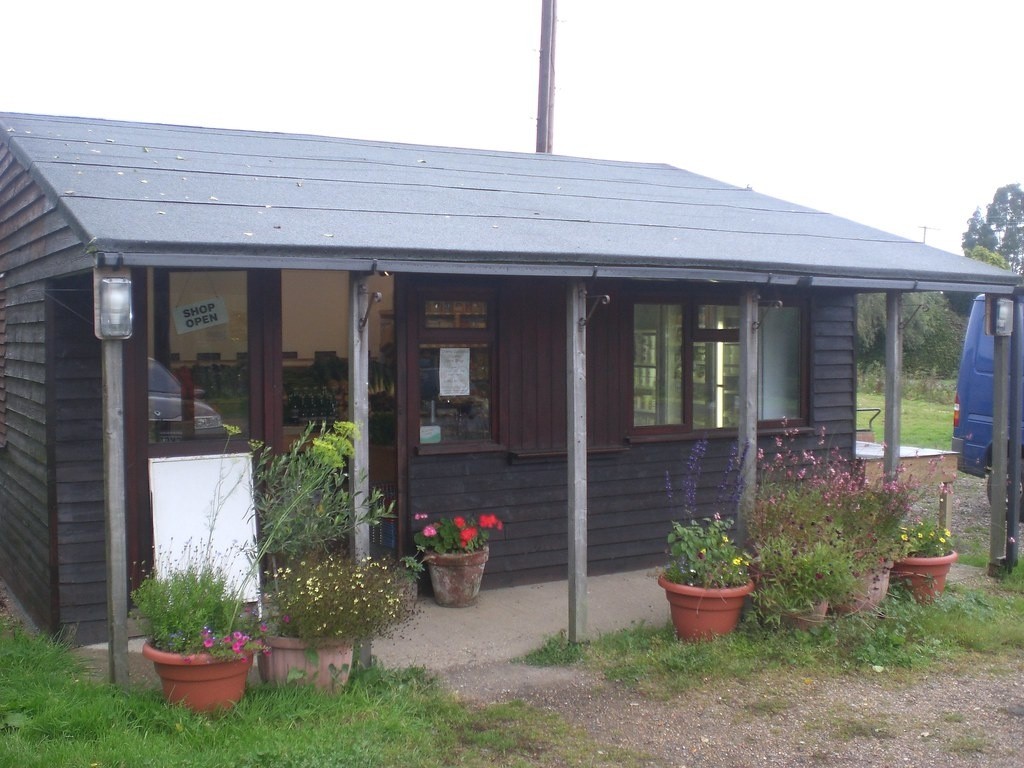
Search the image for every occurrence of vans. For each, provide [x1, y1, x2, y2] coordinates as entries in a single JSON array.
[[949, 295, 1024, 526]]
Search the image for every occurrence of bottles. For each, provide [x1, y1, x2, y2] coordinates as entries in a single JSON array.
[[278, 383, 338, 418]]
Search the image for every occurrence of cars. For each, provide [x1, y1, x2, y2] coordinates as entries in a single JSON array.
[[147, 356, 230, 445]]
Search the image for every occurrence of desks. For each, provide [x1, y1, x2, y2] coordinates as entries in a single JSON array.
[[855, 441, 962, 531]]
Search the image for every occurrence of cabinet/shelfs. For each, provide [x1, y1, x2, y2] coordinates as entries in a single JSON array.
[[169, 300, 490, 450]]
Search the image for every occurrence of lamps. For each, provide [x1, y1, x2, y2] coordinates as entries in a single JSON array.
[[101, 277, 133, 338]]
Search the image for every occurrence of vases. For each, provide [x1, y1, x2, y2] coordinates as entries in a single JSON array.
[[891, 551, 957, 603], [142, 644, 255, 712], [747, 562, 829, 631], [826, 561, 894, 613], [425, 544, 490, 607], [257, 635, 355, 693], [656, 570, 753, 641]]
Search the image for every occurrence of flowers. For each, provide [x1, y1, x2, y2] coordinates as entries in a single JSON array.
[[414, 512, 504, 555], [128, 416, 430, 663], [656, 417, 954, 615]]
